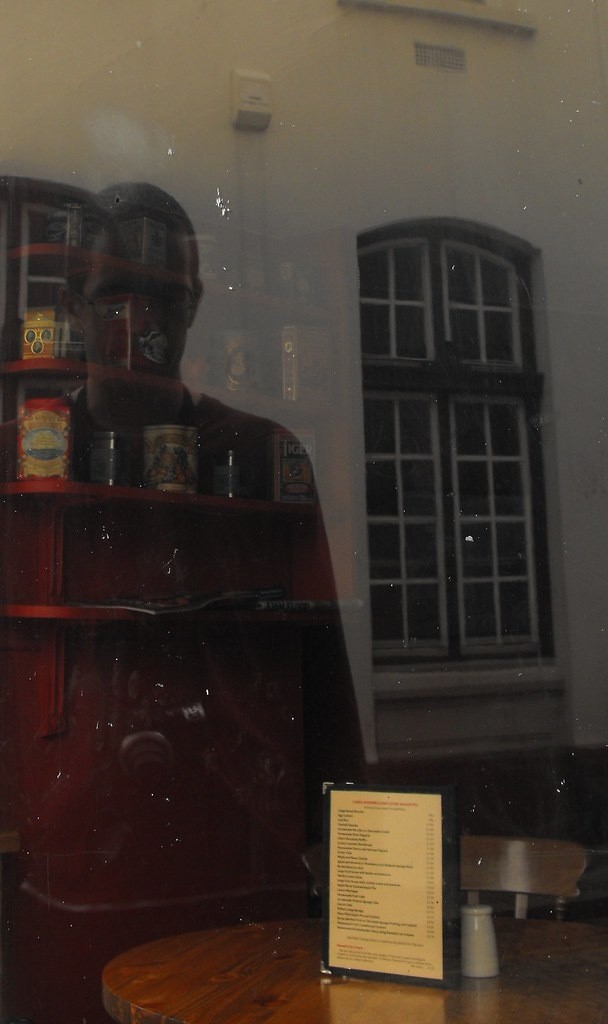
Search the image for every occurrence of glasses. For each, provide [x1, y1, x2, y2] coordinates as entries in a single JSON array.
[[70, 288, 199, 328]]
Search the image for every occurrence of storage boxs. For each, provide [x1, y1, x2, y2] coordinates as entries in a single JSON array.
[[95, 293, 169, 374], [283, 323, 333, 402], [18, 304, 59, 363], [117, 210, 167, 266]]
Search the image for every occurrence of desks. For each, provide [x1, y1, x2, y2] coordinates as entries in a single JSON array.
[[100, 915, 608, 1024]]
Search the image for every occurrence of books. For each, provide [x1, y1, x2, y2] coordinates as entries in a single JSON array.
[[63, 584, 365, 616]]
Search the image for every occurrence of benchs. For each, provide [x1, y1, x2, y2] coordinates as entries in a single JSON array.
[[358, 742, 608, 914]]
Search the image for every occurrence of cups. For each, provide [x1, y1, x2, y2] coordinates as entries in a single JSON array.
[[89, 429, 130, 487], [210, 447, 248, 498]]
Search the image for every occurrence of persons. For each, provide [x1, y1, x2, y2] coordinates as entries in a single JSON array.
[[0, 182, 369, 1024]]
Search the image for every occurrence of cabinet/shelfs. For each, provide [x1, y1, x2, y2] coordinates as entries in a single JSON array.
[[1, 175, 364, 737]]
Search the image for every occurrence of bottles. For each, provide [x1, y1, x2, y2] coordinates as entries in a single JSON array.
[[142, 424, 200, 495], [458, 904, 501, 978]]
